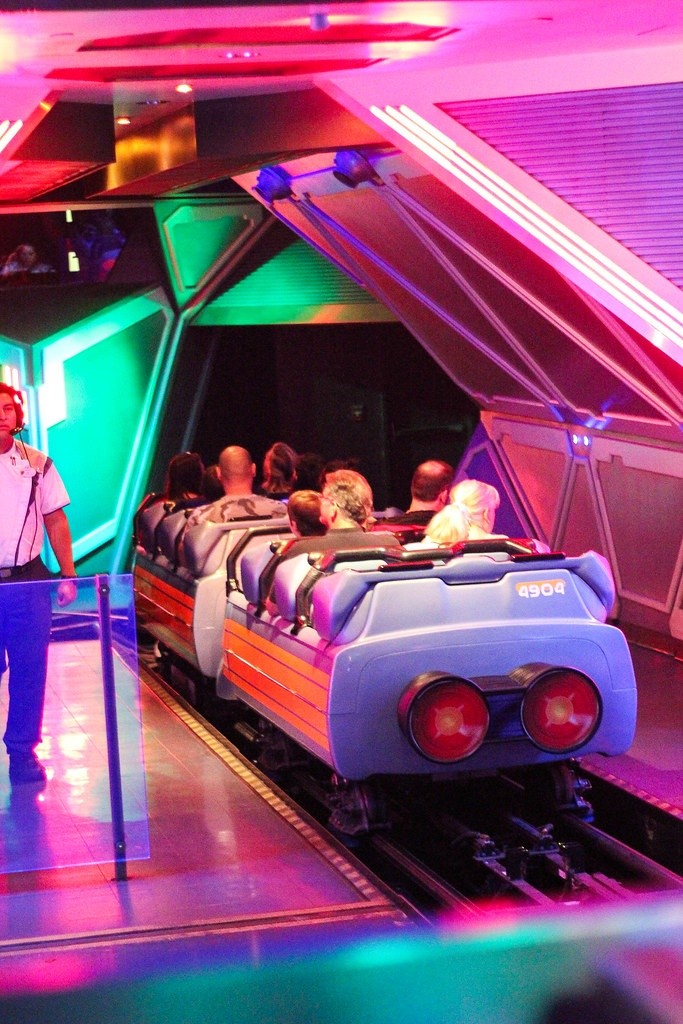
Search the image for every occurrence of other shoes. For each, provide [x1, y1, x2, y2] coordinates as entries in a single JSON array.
[[9, 757, 47, 780]]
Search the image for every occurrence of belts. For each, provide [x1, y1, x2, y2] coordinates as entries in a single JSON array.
[[0, 554, 41, 579]]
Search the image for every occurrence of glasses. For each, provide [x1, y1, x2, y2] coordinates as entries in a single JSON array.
[[317, 491, 334, 508]]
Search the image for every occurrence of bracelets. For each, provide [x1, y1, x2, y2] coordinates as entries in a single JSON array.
[[59, 574, 78, 585]]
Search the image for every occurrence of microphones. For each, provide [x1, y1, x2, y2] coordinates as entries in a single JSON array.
[[10, 422, 22, 434]]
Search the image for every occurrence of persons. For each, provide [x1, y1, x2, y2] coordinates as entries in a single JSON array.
[[318, 470, 373, 536], [287, 490, 329, 538], [0, 382, 80, 783], [135, 449, 368, 515], [3, 244, 56, 276], [176, 445, 289, 569], [69, 210, 124, 284], [261, 442, 303, 502], [417, 478, 510, 544], [372, 458, 454, 526]]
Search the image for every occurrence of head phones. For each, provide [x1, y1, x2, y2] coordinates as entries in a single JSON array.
[[0, 383, 24, 427]]
